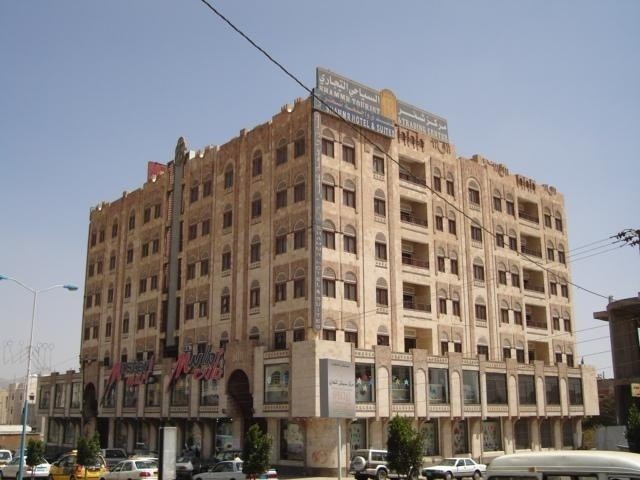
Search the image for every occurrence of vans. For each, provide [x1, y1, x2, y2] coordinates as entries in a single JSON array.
[[486, 451, 640, 480]]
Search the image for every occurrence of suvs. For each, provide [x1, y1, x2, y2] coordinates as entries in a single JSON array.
[[348, 449, 419, 480]]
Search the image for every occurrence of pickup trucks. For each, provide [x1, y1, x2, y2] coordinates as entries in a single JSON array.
[[193, 461, 278, 480]]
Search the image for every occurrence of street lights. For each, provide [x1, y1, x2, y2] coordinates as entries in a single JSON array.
[[1, 274, 79, 479]]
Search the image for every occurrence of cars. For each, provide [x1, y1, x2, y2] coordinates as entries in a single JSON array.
[[1, 449, 51, 479], [422, 457, 486, 480], [52, 448, 193, 480]]
